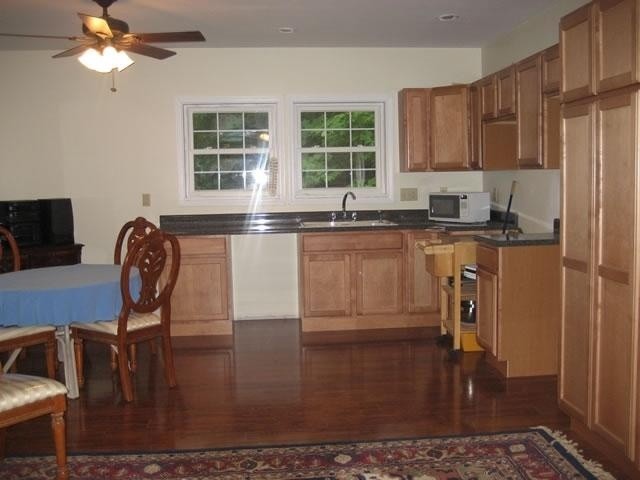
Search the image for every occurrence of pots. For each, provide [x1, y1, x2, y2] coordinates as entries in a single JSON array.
[[460, 300, 475, 323]]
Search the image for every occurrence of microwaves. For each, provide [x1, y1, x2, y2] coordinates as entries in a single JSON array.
[[428, 192, 490, 223]]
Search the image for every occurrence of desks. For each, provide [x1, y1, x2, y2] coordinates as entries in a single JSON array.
[[70, 229, 181, 400], [0, 263, 157, 403]]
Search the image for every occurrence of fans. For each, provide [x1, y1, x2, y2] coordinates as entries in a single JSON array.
[[0, 1, 206, 58]]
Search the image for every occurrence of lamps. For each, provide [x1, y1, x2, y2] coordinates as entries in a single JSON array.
[[79, 0, 135, 92]]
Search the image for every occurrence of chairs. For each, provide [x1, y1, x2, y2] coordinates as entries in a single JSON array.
[[81, 218, 162, 371], [0, 372, 68, 479], [0, 225, 20, 272], [1, 325, 58, 378]]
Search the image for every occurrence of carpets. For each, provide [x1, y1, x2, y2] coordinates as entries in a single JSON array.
[[0, 426, 621, 480]]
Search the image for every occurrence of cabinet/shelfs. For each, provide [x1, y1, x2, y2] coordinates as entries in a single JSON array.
[[406, 220, 443, 340], [430, 84, 469, 171], [159, 223, 233, 338], [555, 84, 639, 463], [542, 46, 560, 95], [483, 114, 516, 169], [469, 78, 482, 170], [483, 62, 515, 120], [399, 88, 430, 171], [471, 231, 561, 378], [298, 220, 406, 333], [560, 0, 640, 105], [515, 52, 542, 169], [409, 233, 474, 358], [542, 93, 560, 169]]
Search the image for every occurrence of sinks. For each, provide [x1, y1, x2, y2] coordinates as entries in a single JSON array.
[[303, 219, 397, 228]]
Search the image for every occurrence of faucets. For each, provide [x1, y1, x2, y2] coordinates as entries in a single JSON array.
[[342, 191, 356, 217]]
